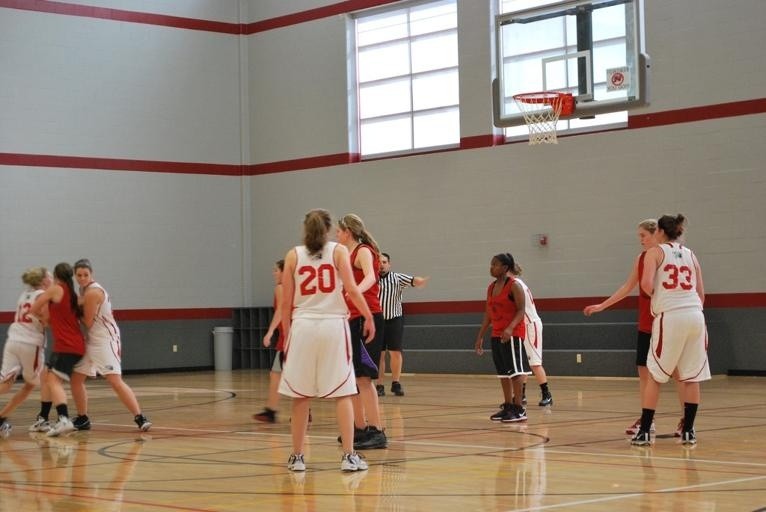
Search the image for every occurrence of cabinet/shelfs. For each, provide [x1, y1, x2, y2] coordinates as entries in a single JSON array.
[[233, 306, 277, 370]]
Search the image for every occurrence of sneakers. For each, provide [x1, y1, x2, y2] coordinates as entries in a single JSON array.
[[341, 452, 368, 471], [252, 407, 276, 422], [674, 419, 697, 445], [626, 415, 656, 446], [338, 421, 388, 449], [539, 392, 552, 406], [490, 396, 528, 423], [135, 413, 152, 432], [28, 415, 91, 436], [287, 454, 306, 471], [376, 384, 404, 396]]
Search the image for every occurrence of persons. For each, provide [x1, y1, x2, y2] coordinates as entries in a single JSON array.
[[69, 258, 152, 431], [631, 438, 715, 511], [635, 443, 686, 511], [251, 260, 313, 426], [286, 394, 410, 512], [375, 252, 432, 396], [281, 209, 376, 472], [515, 408, 554, 511], [335, 213, 388, 449], [584, 218, 698, 437], [0, 432, 154, 512], [473, 252, 534, 423], [0, 267, 53, 431], [27, 262, 87, 436], [627, 213, 714, 445], [478, 419, 532, 511], [499, 263, 553, 408]]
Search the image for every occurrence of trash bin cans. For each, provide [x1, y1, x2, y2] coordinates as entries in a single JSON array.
[[211, 326, 234, 370]]
